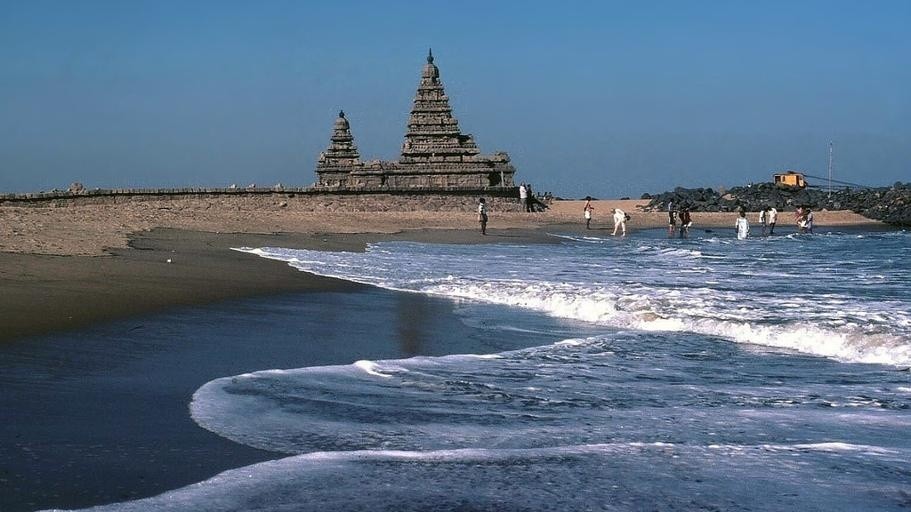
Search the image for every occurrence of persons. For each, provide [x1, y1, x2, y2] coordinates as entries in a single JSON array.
[[791, 203, 815, 235], [734, 211, 750, 240], [767, 206, 778, 235], [667, 197, 695, 239], [515, 180, 556, 214], [609, 207, 630, 238], [477, 198, 488, 235], [758, 206, 768, 233], [583, 196, 595, 229]]
